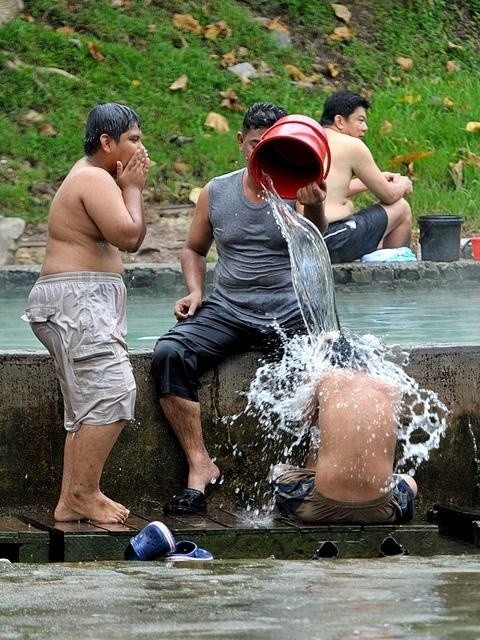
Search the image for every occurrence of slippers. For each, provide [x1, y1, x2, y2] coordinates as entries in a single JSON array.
[[126, 520, 174, 567], [163, 540, 215, 562], [163, 484, 215, 520]]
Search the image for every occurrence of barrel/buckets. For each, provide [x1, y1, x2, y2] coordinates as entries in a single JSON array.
[[416, 214, 464, 262], [468, 237, 480, 260], [248, 114, 331, 200]]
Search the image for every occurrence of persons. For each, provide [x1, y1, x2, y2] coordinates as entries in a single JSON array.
[[267, 334, 419, 526], [317, 89, 414, 264], [149, 103, 374, 516], [19, 103, 153, 525]]
